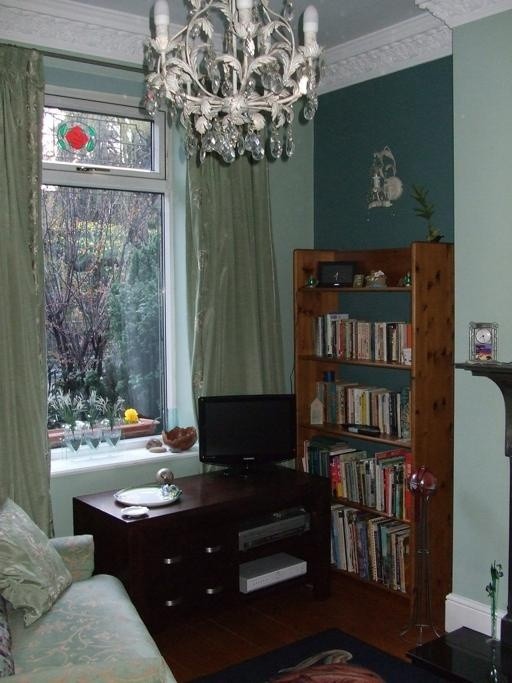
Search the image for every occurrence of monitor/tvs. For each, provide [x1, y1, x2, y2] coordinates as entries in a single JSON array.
[[198, 394, 297, 480]]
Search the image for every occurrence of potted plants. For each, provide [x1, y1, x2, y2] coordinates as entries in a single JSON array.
[[102, 398, 125, 448], [51, 398, 84, 453], [80, 394, 104, 449], [412, 183, 445, 242], [484, 559, 504, 681]]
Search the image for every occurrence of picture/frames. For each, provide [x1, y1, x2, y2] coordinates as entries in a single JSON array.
[[316, 261, 356, 287]]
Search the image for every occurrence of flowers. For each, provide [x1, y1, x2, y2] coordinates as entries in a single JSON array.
[[124, 408, 138, 422]]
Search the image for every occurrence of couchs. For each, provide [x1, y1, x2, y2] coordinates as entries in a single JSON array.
[[0, 531, 177, 683]]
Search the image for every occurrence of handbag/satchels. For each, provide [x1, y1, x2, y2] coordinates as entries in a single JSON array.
[[269, 649, 384, 682]]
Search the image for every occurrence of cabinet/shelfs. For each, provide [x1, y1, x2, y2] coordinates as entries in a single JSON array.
[[293, 243, 455, 618]]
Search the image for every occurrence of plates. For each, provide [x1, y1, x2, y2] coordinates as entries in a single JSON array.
[[113, 485, 181, 507], [120, 506, 149, 518]]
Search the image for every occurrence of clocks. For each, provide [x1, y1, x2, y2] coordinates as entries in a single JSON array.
[[476, 327, 492, 343]]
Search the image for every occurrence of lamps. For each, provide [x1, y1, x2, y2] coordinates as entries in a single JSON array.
[[143, 0, 324, 163]]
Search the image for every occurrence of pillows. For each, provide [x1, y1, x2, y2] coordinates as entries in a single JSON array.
[[1, 497, 73, 629]]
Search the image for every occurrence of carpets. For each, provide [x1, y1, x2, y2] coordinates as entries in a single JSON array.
[[188, 626, 413, 682]]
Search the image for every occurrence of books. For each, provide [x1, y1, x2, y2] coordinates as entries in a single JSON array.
[[300, 312, 413, 595]]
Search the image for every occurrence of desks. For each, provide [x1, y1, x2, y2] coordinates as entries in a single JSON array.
[[408, 626, 501, 682], [73, 464, 331, 623]]
